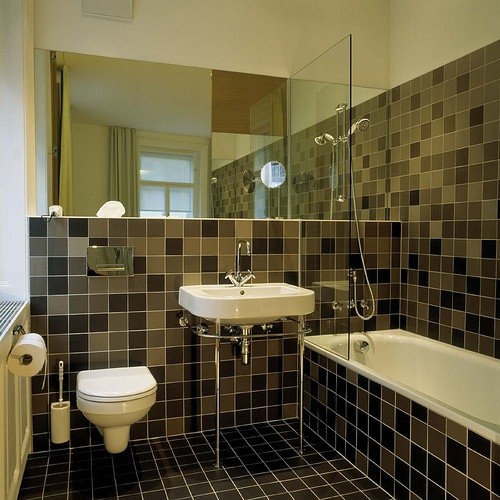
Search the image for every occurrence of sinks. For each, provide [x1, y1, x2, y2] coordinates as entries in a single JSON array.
[[179, 283, 315, 326]]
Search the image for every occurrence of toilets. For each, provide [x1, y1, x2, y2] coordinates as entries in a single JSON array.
[[76, 366, 158, 454]]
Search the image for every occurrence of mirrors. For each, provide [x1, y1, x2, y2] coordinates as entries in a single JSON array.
[[261, 161, 287, 220], [243, 169, 275, 220], [34, 48, 389, 219]]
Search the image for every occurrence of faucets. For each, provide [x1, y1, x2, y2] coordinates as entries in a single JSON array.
[[224, 240, 256, 286]]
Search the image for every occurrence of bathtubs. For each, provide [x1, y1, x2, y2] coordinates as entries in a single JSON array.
[[305, 328, 500, 446]]
